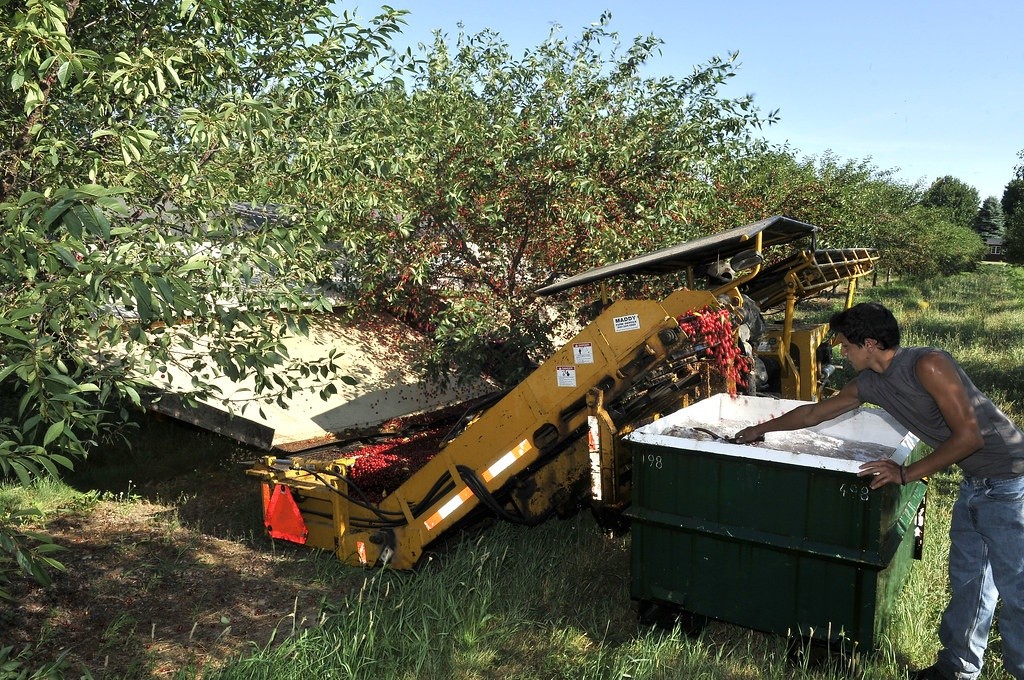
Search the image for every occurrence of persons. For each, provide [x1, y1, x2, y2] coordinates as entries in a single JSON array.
[[733, 303, 1024, 680]]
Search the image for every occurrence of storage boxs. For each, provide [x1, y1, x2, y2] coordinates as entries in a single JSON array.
[[626, 392, 921, 567], [631, 518, 924, 654]]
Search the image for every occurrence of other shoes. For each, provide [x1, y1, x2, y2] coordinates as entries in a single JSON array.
[[911, 664, 948, 680]]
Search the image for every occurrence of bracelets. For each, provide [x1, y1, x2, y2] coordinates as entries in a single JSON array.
[[900, 465, 907, 487]]
